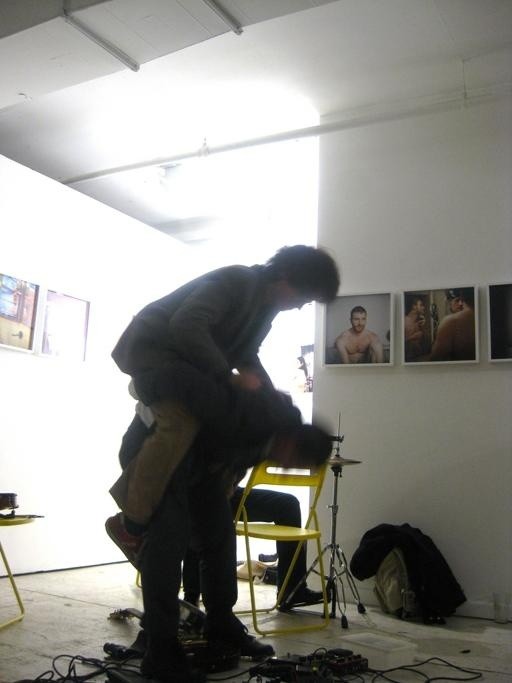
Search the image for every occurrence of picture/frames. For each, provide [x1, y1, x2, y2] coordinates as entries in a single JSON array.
[[0, 272, 92, 365], [320, 282, 511, 367]]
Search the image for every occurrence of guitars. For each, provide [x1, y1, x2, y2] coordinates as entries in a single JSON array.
[[109, 607, 240, 673]]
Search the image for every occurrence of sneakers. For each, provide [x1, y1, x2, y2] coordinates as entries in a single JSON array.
[[105, 512, 143, 572], [277, 588, 323, 612]]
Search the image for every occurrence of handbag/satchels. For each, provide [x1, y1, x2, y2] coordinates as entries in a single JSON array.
[[373, 546, 447, 625]]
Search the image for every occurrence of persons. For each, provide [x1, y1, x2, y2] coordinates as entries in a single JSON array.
[[104, 244, 343, 576], [334, 304, 384, 363], [405, 287, 475, 361], [233, 486, 328, 612], [107, 351, 332, 682]]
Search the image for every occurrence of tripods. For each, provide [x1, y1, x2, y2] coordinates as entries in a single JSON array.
[[281, 471, 365, 630]]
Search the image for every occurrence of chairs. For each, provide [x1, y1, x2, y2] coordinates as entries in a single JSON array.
[[232, 443, 331, 634]]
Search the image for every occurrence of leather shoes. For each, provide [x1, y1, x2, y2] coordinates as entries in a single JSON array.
[[140, 635, 207, 682], [204, 613, 276, 657]]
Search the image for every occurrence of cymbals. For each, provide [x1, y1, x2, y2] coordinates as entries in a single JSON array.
[[326, 454, 362, 467]]
